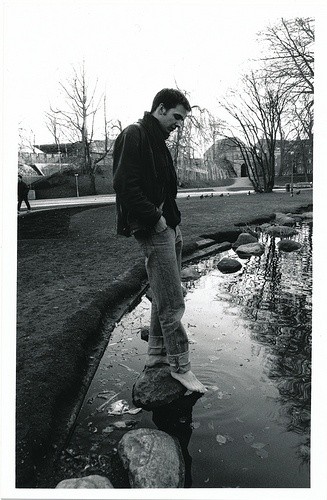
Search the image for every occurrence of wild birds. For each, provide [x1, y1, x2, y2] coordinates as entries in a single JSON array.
[[247, 191, 251, 196], [186, 193, 230, 200], [289, 190, 301, 197]]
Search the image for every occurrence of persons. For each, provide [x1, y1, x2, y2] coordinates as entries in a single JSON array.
[[17, 175, 31, 214], [113, 88, 208, 395]]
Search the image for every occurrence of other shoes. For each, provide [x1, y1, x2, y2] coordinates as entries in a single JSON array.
[[27, 210, 31, 213], [18, 211, 20, 214]]
